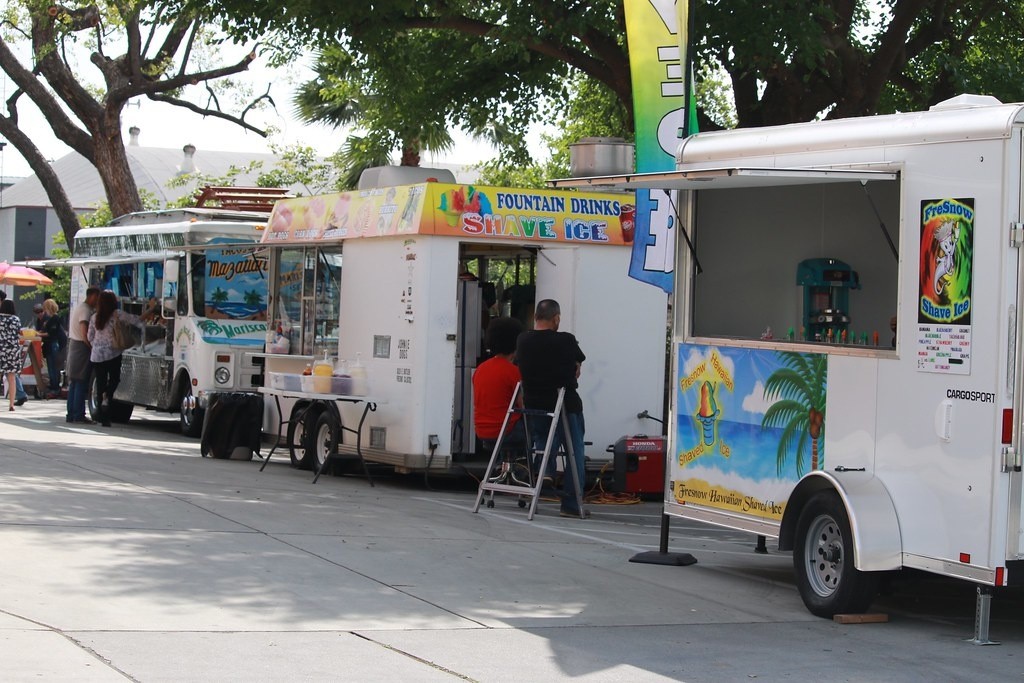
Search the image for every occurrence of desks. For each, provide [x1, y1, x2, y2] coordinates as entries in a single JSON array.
[[257, 387, 381, 488]]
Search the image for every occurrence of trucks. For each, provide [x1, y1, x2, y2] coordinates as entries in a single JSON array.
[[9, 208, 302, 437]]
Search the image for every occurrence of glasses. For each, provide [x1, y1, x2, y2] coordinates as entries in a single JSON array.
[[36, 310, 43, 314]]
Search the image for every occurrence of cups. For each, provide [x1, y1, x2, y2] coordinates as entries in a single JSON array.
[[619, 205, 635, 242]]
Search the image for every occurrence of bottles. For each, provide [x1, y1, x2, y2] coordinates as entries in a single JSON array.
[[265, 320, 288, 342], [787, 326, 878, 346], [302, 349, 368, 397]]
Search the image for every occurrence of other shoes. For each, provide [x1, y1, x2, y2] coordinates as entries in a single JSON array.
[[541, 474, 556, 497], [8, 407, 15, 411], [560, 509, 591, 518], [14, 395, 28, 406], [67, 418, 97, 425]]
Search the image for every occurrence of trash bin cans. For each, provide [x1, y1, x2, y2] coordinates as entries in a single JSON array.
[[210, 393, 264, 461]]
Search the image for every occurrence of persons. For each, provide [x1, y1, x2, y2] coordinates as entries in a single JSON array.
[[65, 287, 99, 425], [0, 289, 29, 411], [32, 300, 64, 399], [88, 289, 157, 427], [514, 299, 590, 517], [472, 317, 529, 485]]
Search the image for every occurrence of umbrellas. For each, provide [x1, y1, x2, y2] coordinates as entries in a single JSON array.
[[0, 262, 53, 293]]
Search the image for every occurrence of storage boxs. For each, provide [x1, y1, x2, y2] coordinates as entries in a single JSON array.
[[297, 374, 371, 397], [268, 371, 301, 391], [266, 343, 291, 355]]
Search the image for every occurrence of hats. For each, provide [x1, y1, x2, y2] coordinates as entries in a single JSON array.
[[33, 304, 43, 312]]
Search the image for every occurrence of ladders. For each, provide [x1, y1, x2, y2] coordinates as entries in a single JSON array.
[[472, 381, 587, 521]]
